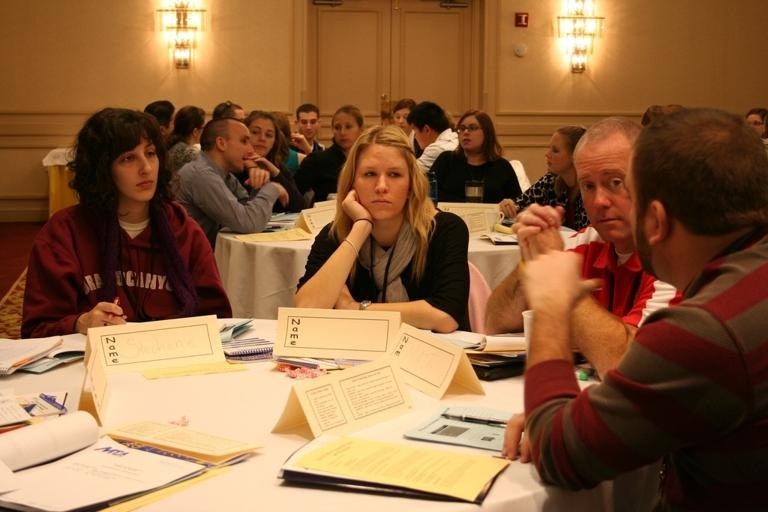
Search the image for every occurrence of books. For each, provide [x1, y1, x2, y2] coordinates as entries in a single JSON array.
[[0, 333, 89, 435]]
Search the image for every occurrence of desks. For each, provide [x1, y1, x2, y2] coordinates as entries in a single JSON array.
[[213, 194, 584, 322], [0, 315, 659, 511]]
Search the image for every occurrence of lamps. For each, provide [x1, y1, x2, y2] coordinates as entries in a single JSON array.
[[152, 0, 205, 67], [555, 0, 604, 74]]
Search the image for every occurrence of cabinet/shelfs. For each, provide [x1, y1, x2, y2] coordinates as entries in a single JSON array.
[[43, 147, 81, 220]]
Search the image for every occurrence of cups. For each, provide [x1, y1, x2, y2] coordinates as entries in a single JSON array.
[[485, 209, 505, 231], [521, 309, 534, 363]]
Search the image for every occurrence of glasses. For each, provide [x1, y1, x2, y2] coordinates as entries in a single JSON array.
[[456, 123, 477, 134]]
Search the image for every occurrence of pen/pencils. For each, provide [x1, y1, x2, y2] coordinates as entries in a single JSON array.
[[262, 225, 283, 232], [104, 297, 120, 326], [441, 414, 508, 425], [59, 393, 68, 416]]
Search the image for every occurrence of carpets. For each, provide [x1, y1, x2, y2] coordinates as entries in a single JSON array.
[[0, 266, 29, 340]]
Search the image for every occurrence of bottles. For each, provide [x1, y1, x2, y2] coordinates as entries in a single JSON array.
[[422, 171, 439, 208]]
